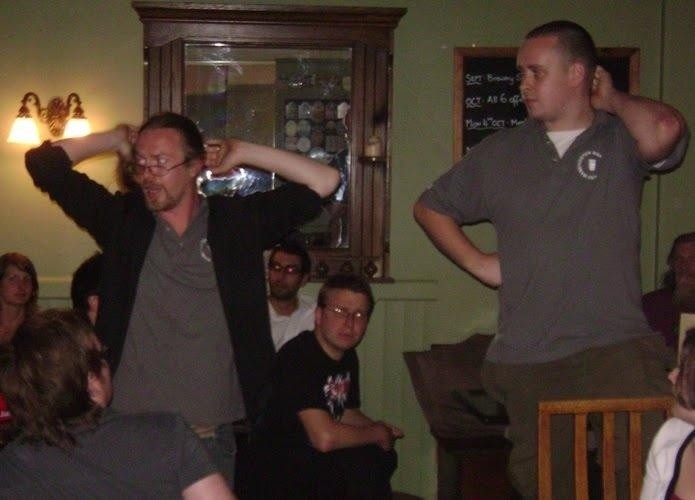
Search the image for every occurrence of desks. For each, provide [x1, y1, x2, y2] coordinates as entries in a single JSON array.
[[402, 333, 527, 500]]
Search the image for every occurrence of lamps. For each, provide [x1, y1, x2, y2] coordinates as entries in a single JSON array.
[[4, 90, 92, 147]]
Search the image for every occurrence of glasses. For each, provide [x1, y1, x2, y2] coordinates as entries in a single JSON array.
[[126, 160, 189, 178]]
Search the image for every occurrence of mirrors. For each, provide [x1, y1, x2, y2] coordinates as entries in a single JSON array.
[[185, 43, 356, 247]]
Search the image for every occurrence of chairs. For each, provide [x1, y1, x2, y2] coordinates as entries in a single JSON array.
[[536, 393, 675, 500]]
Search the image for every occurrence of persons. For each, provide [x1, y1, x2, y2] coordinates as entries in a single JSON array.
[[238, 274, 406, 499], [68, 250, 103, 329], [639, 327, 695, 500], [412, 17, 690, 500], [640, 231, 695, 346], [0, 252, 40, 428], [0, 304, 237, 500], [25, 110, 342, 499], [262, 242, 319, 352]]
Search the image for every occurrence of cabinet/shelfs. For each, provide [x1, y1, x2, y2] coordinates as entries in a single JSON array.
[[284, 98, 350, 156]]
